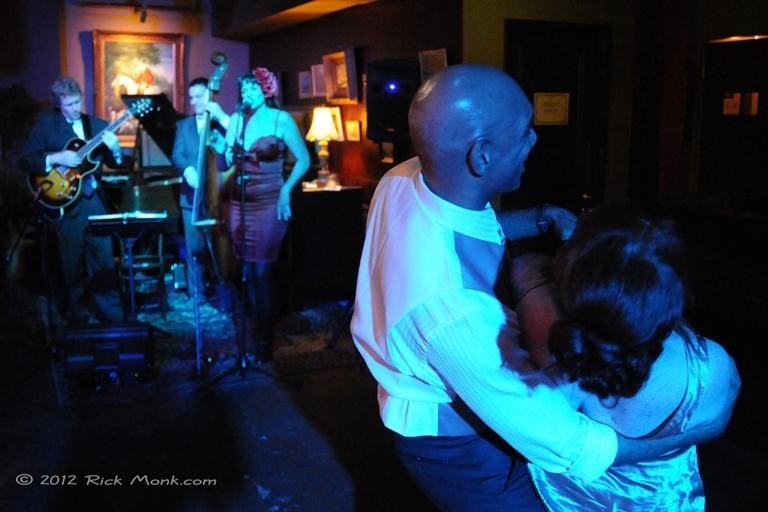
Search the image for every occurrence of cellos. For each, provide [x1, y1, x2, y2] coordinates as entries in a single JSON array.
[[191, 50, 233, 293]]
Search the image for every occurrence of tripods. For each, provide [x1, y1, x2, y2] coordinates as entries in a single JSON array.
[[189, 116, 302, 397], [127, 229, 135, 328]]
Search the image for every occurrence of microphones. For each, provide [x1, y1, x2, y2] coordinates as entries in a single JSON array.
[[238, 98, 253, 112]]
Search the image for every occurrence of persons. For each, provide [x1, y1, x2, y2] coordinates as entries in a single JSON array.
[[350, 64, 578, 512], [172, 76, 231, 305], [503, 237, 740, 512], [206, 67, 310, 364], [19, 77, 123, 318]]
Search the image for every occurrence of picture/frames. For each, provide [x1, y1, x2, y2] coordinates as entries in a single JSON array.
[[298, 72, 311, 98], [311, 65, 326, 97], [92, 29, 185, 149], [321, 49, 358, 103]]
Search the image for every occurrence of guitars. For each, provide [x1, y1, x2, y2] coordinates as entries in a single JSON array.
[[26, 98, 153, 209]]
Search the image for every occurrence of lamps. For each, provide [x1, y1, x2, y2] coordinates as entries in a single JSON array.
[[305, 106, 338, 185]]
